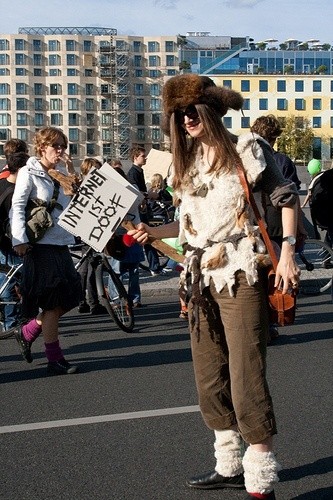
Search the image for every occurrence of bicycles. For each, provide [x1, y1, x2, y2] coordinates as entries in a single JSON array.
[[296, 189, 333, 297], [0, 237, 135, 339], [137, 201, 180, 270]]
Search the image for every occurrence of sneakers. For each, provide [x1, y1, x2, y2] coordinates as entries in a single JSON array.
[[48, 359, 79, 374], [90, 304, 109, 314], [78, 304, 90, 313], [14, 326, 33, 363]]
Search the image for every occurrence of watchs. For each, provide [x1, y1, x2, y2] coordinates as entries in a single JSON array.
[[282, 235, 295, 244]]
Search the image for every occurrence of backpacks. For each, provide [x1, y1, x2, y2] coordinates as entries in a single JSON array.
[[262, 192, 306, 253], [147, 198, 172, 227], [308, 168, 333, 231]]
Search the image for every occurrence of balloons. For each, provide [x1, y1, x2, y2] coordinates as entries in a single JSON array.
[[307, 158, 321, 174]]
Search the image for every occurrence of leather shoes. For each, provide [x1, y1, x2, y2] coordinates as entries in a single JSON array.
[[245, 490, 276, 500], [187, 470, 245, 488]]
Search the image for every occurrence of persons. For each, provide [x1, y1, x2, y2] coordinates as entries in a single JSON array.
[[11, 128, 78, 375], [0, 116, 333, 344], [127, 73, 302, 500]]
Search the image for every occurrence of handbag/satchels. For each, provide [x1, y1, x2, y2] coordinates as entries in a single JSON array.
[[268, 268, 296, 327], [24, 198, 55, 242]]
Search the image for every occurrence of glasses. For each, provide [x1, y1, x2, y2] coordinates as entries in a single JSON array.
[[174, 104, 199, 124], [48, 143, 67, 150]]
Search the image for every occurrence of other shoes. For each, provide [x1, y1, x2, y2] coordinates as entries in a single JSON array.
[[267, 328, 279, 346], [152, 270, 168, 277], [179, 311, 189, 318], [321, 262, 333, 269], [111, 303, 121, 310], [133, 303, 141, 308]]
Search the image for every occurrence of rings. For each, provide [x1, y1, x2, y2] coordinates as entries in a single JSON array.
[[292, 283, 298, 287]]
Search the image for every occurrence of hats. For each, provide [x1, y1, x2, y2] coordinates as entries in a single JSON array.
[[307, 158, 321, 174], [161, 74, 254, 139]]
